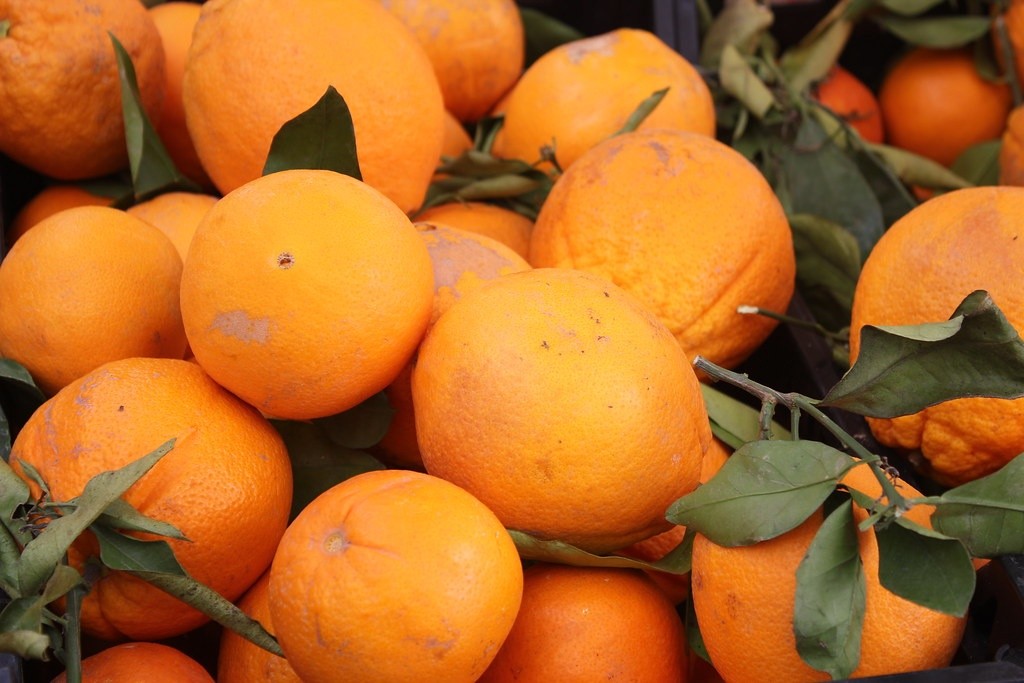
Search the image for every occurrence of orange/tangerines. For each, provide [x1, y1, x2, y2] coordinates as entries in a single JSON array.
[[0, 0, 1024, 683]]
